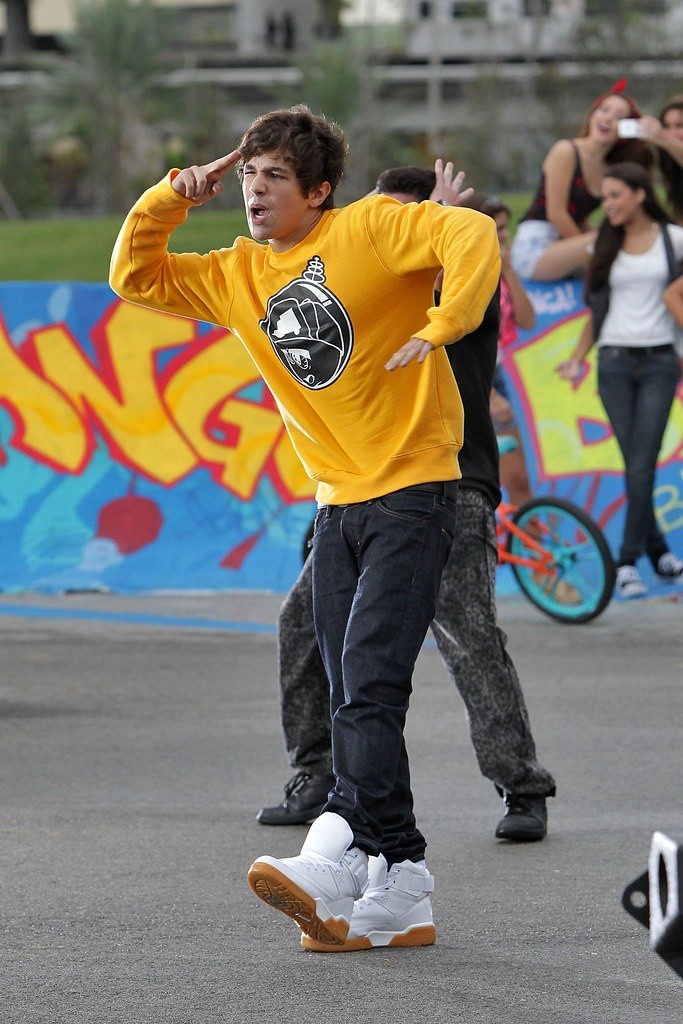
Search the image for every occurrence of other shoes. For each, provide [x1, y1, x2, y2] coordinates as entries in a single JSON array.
[[656, 552, 683, 574], [613, 564, 649, 599]]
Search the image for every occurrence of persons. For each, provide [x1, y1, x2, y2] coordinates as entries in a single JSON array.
[[255, 156, 558, 842], [108, 109, 502, 953], [462, 80, 683, 599]]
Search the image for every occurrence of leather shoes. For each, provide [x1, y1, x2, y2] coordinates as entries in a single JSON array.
[[495, 795, 548, 838], [254, 762, 336, 824]]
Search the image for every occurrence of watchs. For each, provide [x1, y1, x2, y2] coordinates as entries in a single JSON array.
[[436, 199, 450, 207]]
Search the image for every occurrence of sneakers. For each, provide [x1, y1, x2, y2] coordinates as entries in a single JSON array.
[[246, 811, 369, 947], [300, 852, 436, 952]]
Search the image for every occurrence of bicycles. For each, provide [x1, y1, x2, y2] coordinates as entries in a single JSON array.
[[301, 435, 617, 630]]
